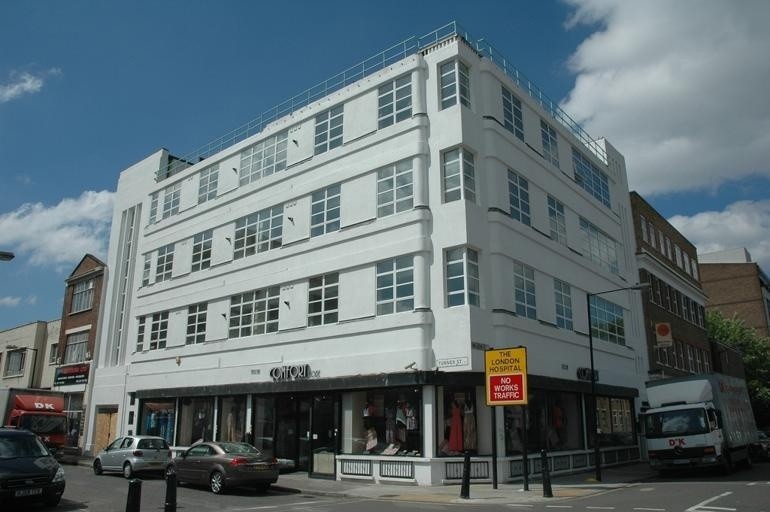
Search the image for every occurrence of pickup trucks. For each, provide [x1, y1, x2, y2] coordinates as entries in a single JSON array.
[[0, 387, 73, 461]]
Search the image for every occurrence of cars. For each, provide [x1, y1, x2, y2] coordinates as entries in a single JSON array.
[[748, 427, 770, 462], [163, 441, 280, 496], [93, 435, 172, 479]]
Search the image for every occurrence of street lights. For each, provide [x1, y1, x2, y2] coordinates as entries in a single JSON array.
[[586, 281, 652, 481]]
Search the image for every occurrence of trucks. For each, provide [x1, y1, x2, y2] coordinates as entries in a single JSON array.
[[636, 372, 761, 479]]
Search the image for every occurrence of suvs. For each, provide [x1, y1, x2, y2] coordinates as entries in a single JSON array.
[[0, 426, 66, 512]]
